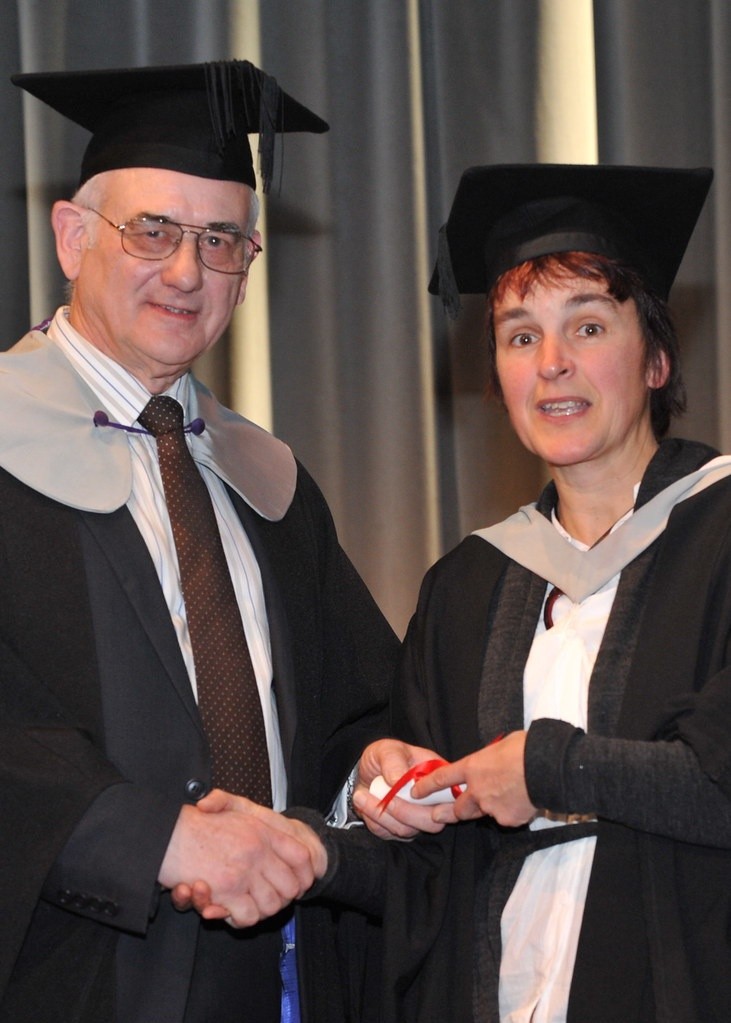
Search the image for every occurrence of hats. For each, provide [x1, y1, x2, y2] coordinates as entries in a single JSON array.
[[9, 59, 331, 197], [425, 163, 717, 323]]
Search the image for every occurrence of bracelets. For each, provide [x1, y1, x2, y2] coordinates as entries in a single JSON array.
[[347, 796, 360, 820]]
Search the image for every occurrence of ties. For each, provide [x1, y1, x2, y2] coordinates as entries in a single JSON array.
[[142, 393, 272, 809]]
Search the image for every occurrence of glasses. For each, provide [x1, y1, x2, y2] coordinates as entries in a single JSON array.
[[83, 207, 262, 276]]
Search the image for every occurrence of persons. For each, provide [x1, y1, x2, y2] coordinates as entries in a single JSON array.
[[2, 56, 731, 1023]]
